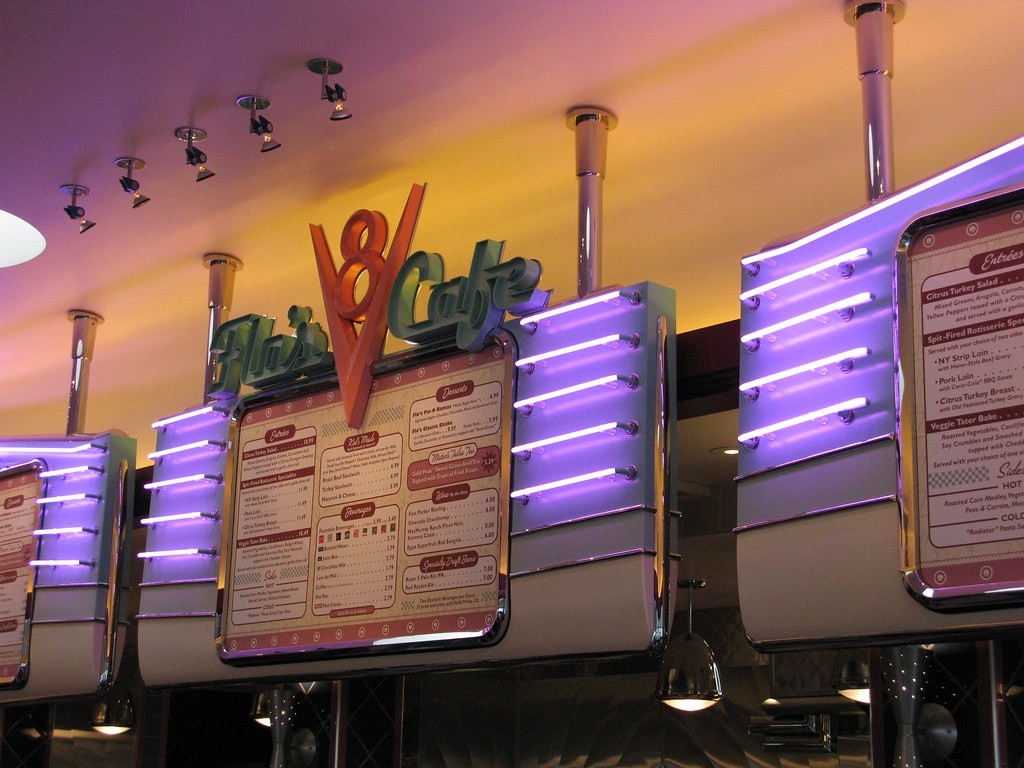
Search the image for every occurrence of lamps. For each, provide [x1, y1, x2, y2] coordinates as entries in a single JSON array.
[[307, 59, 355, 121], [658, 588, 721, 711], [112, 157, 151, 209], [249, 687, 290, 727], [91, 692, 135, 735], [175, 127, 217, 183], [837, 651, 868, 690], [59, 185, 97, 235], [237, 96, 283, 152]]
